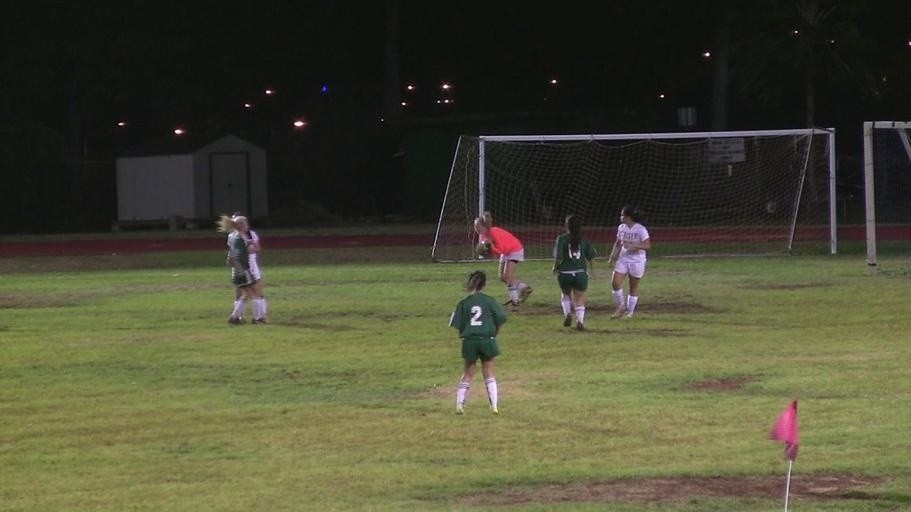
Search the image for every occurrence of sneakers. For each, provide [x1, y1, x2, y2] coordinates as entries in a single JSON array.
[[502, 286, 533, 308], [226, 317, 271, 325], [610, 306, 635, 319], [564, 313, 585, 330]]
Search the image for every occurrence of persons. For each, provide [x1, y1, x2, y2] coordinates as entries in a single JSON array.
[[551, 213, 599, 333], [474, 212, 533, 307], [216, 213, 257, 325], [229, 215, 272, 325], [448, 271, 508, 417], [607, 207, 652, 321]]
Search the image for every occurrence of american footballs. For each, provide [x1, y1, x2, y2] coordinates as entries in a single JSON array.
[[477, 240, 490, 256]]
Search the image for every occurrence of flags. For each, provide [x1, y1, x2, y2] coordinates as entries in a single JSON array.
[[769, 400, 804, 464]]
